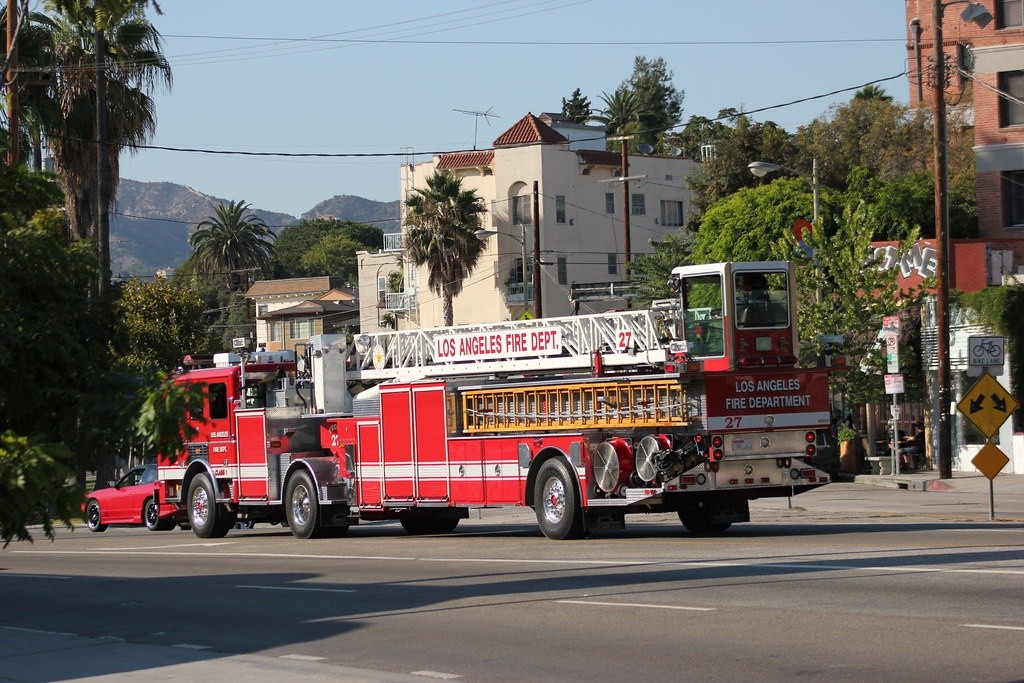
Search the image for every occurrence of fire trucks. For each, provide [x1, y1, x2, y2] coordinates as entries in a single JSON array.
[[154, 259, 837, 544]]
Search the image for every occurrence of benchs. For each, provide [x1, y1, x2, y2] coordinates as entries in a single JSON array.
[[862, 455, 897, 476]]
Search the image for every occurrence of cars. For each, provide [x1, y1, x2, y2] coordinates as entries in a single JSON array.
[[80, 462, 192, 530]]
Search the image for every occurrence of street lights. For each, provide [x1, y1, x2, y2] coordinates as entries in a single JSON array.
[[473, 224, 529, 314], [748, 156, 824, 364], [933, 0, 995, 478]]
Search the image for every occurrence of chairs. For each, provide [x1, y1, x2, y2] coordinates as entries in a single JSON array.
[[874, 438, 935, 472]]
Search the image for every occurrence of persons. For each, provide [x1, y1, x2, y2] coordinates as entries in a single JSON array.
[[898, 422, 926, 471]]
